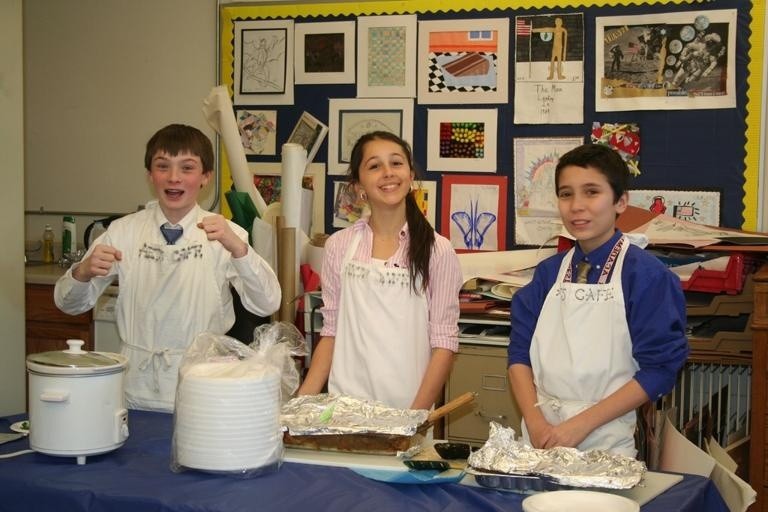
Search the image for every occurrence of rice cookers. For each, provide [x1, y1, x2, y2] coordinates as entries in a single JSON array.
[[25, 337, 129, 467]]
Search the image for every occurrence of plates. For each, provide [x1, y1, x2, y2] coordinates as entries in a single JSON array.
[[9, 421, 30, 436], [173, 362, 284, 471]]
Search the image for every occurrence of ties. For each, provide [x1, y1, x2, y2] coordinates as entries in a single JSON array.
[[160, 222, 183, 245], [576, 260, 592, 284]]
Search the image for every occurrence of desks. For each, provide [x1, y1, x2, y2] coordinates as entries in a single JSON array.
[[24, 237, 120, 353]]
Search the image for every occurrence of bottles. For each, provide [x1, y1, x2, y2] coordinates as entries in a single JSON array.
[[42, 222, 55, 264]]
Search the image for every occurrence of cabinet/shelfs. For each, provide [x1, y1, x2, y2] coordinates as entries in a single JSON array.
[[444, 336, 524, 449]]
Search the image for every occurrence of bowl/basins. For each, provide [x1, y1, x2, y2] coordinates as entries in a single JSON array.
[[521, 490, 641, 512]]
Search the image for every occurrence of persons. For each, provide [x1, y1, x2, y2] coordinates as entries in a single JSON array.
[[52, 120, 285, 413], [293, 129, 465, 439], [505, 141, 689, 464]]
